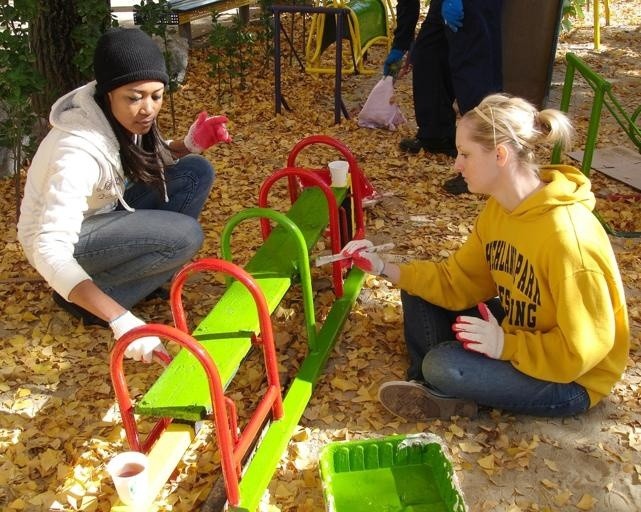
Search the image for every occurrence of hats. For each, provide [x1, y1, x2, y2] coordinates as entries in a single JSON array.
[[95, 28, 169, 93]]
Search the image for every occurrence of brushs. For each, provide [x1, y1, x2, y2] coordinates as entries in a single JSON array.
[[311, 242, 395, 268]]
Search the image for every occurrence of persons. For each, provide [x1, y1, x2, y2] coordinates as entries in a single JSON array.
[[17, 29, 231, 366], [385, 0, 502, 194], [340, 95, 631, 423]]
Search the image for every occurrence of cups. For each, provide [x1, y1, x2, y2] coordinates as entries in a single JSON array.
[[106, 451, 154, 503], [328, 160, 350, 187]]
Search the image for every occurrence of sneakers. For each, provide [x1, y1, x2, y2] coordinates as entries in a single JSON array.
[[443, 172, 471, 194], [399, 138, 457, 158], [53, 291, 108, 328], [378, 380, 477, 425], [146, 287, 184, 301]]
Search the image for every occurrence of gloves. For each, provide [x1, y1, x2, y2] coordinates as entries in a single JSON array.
[[453, 303, 504, 360], [184, 112, 232, 154], [384, 49, 403, 79], [442, 1, 464, 32], [108, 311, 171, 363], [339, 239, 384, 277]]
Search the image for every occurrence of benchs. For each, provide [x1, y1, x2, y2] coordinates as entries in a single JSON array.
[[133, 0, 250, 45]]
